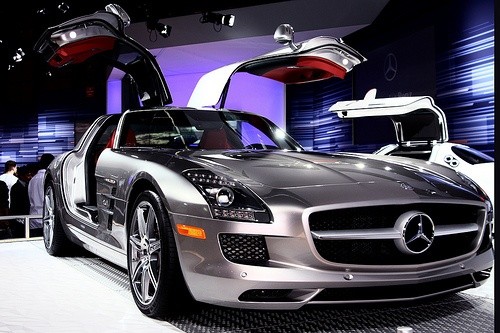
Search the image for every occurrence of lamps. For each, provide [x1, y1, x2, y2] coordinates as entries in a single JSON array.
[[146, 9, 172, 42], [199, 12, 235, 32]]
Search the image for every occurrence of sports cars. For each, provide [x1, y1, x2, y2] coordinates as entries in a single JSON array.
[[33, 3, 494, 318], [327, 89, 494, 221]]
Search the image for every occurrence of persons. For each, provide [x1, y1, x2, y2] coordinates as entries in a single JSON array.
[[0, 160, 34, 239], [29, 153, 55, 237]]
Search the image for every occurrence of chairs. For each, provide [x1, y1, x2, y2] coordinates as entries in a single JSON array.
[[96, 129, 136, 164], [199, 129, 227, 149]]
